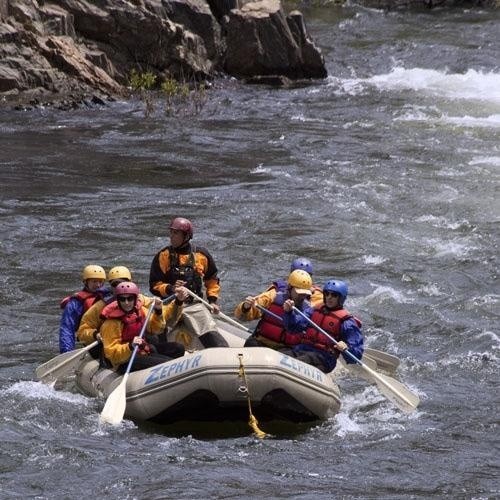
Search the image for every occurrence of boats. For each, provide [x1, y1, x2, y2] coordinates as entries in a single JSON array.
[[75, 319, 341, 422]]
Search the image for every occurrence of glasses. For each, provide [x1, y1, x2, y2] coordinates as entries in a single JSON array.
[[110, 280, 123, 288], [323, 291, 342, 299], [117, 295, 136, 302]]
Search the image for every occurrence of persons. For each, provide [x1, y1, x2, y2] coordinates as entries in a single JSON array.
[[265, 257, 324, 306], [59, 265, 107, 353], [234, 270, 312, 351], [283, 280, 364, 373], [99, 282, 173, 373], [76, 265, 189, 360], [150, 217, 229, 348]]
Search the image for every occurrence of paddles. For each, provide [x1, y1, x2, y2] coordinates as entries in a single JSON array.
[[253, 302, 399, 379], [100, 296, 156, 426], [35, 292, 179, 382], [291, 306, 419, 413], [335, 349, 377, 383]]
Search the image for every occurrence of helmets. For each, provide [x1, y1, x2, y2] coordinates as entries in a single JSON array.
[[114, 281, 139, 308], [107, 265, 132, 282], [290, 257, 313, 275], [323, 278, 348, 306], [287, 269, 313, 290], [81, 264, 107, 283], [167, 217, 194, 238]]
